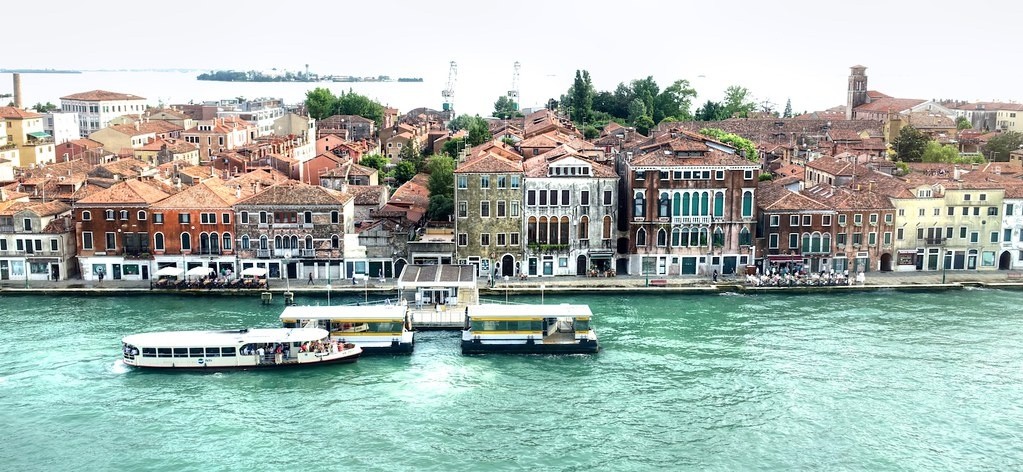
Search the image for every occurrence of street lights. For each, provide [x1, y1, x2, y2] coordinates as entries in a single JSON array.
[[325, 284, 332, 306], [504, 275, 510, 305], [362, 276, 370, 306], [540, 285, 546, 305]]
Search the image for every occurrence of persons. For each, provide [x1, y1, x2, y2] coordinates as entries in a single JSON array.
[[746, 265, 864, 286], [351, 271, 356, 285], [154, 266, 269, 289], [98, 269, 104, 283], [241, 343, 290, 361], [487, 273, 491, 285], [53, 269, 58, 282], [308, 273, 314, 285], [299, 339, 345, 354]]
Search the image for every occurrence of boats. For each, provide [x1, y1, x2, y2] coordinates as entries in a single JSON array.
[[278, 303, 415, 354], [459, 301, 600, 355], [120, 327, 363, 372]]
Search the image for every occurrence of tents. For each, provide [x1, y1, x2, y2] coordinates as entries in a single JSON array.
[[239, 267, 266, 279], [155, 266, 184, 277], [185, 267, 214, 276]]
